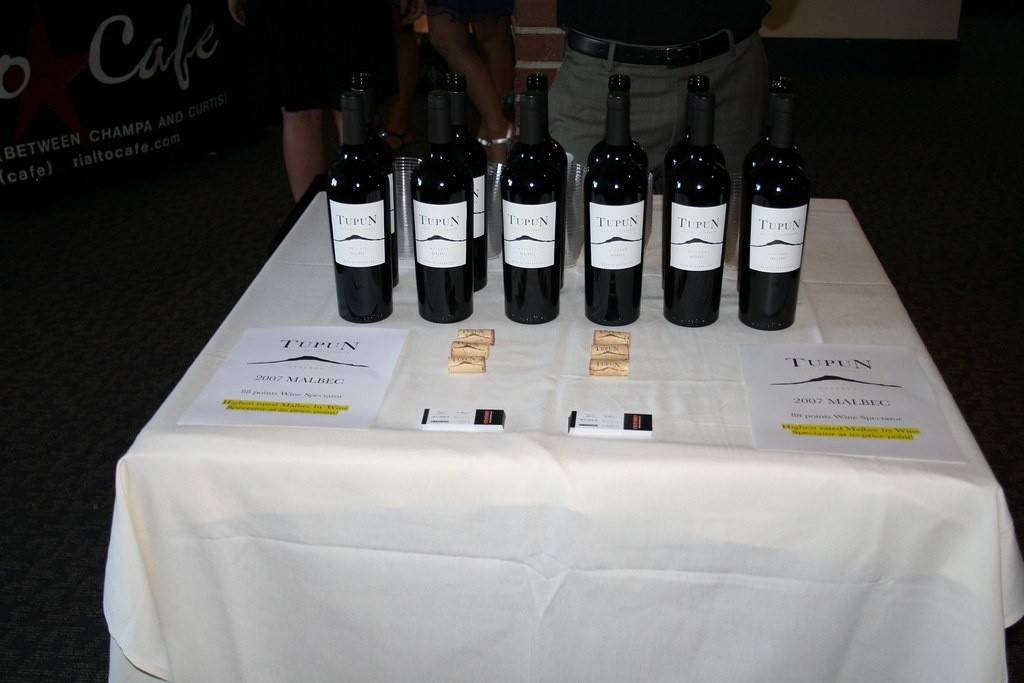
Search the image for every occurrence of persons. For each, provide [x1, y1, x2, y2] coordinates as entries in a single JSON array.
[[226, 0, 424, 203], [424, 0, 516, 163], [547, 0, 797, 194]]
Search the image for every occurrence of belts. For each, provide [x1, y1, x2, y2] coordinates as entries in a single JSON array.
[[566, 16, 761, 69]]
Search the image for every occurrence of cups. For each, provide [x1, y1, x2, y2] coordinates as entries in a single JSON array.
[[724, 171, 742, 268], [488, 162, 505, 260], [565, 163, 586, 264], [395, 157, 423, 256], [644, 171, 652, 253]]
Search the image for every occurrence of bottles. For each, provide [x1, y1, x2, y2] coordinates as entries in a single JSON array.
[[663, 76, 731, 327], [350, 71, 399, 289], [410, 90, 473, 324], [326, 92, 392, 323], [501, 74, 567, 324], [445, 73, 488, 291], [584, 75, 648, 326], [737, 76, 810, 330]]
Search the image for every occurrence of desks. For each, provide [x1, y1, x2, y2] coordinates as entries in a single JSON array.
[[104, 190, 1024, 683]]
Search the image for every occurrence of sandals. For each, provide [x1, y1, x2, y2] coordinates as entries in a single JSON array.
[[478, 123, 513, 178], [381, 127, 413, 151]]
